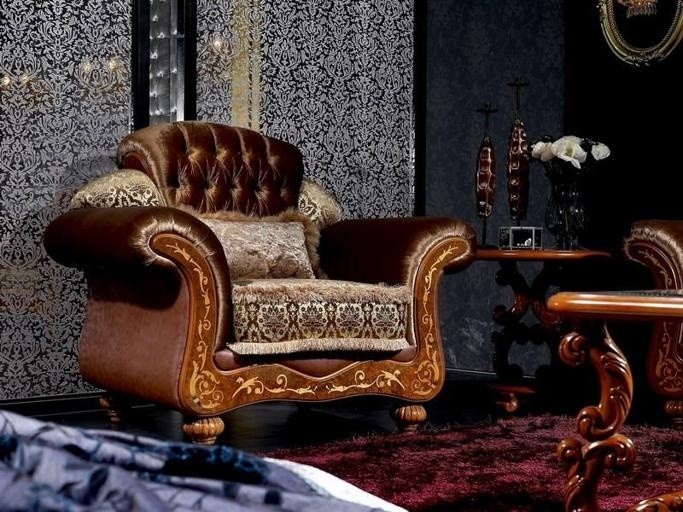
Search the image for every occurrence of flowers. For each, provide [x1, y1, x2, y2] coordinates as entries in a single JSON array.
[[529, 131, 610, 211]]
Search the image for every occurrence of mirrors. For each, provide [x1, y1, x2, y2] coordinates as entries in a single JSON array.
[[595, 0, 683, 66]]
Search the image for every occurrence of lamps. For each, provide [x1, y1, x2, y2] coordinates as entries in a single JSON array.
[[618, 0, 658, 19]]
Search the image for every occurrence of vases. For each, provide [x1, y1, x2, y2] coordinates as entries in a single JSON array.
[[543, 173, 589, 252]]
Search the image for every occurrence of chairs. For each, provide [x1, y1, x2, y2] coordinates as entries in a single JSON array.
[[43, 120, 477, 445], [624, 219, 683, 431]]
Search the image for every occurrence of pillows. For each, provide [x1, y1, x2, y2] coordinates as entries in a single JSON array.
[[184, 205, 326, 281]]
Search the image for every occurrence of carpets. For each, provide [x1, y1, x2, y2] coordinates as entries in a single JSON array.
[[258, 414, 682, 512]]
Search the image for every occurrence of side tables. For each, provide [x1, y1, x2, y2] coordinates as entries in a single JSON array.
[[470, 246, 611, 412]]
